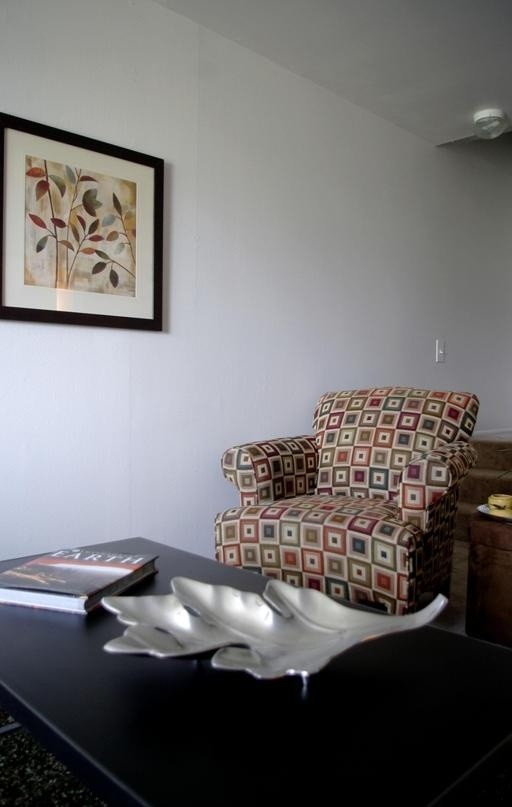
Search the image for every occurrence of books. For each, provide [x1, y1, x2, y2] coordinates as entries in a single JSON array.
[[1, 544, 162, 617]]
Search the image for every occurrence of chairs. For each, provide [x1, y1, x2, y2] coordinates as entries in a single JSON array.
[[212, 381, 484, 625]]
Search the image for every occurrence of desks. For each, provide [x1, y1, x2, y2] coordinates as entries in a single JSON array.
[[2, 529, 511, 802]]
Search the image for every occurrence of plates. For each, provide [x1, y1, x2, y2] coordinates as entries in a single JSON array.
[[477, 502, 512, 520]]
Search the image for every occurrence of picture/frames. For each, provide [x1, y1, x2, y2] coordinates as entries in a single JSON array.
[[1, 108, 166, 334]]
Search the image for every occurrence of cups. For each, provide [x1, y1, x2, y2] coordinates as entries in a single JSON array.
[[487, 493, 512, 509]]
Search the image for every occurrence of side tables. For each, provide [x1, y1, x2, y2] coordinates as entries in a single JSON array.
[[460, 495, 511, 653]]
